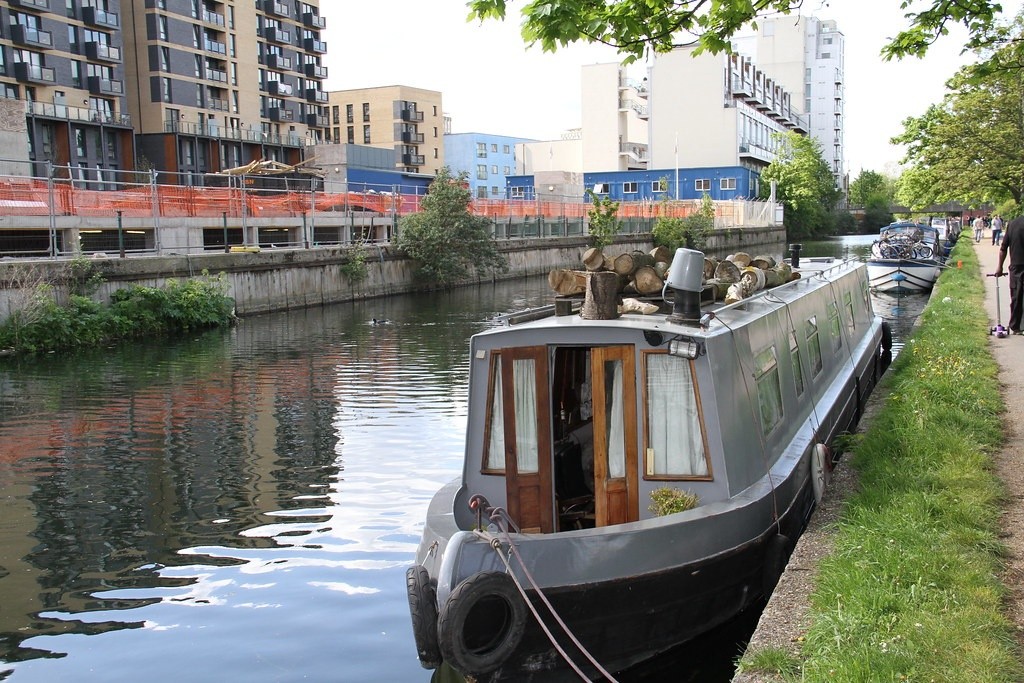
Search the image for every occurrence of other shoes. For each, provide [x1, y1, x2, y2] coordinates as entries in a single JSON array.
[[1013, 331, 1023, 335]]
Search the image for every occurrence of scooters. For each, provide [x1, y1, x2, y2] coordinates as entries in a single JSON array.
[[986, 272, 1012, 338]]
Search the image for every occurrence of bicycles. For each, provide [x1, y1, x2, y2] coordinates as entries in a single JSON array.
[[870, 227, 933, 260]]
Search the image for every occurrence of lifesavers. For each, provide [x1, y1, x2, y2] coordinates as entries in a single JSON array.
[[436, 569, 528, 676], [405, 565, 443, 670]]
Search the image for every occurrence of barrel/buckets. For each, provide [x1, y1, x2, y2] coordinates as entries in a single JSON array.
[[662, 247, 705, 306]]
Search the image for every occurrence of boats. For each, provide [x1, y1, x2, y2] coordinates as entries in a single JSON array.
[[869, 290, 930, 326], [867, 222, 945, 294], [931, 218, 961, 251], [405, 248, 892, 683]]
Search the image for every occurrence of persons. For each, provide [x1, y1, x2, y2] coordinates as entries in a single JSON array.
[[970, 215, 992, 242], [992, 214, 1001, 246], [996, 215, 1024, 336], [998, 215, 1004, 227]]
